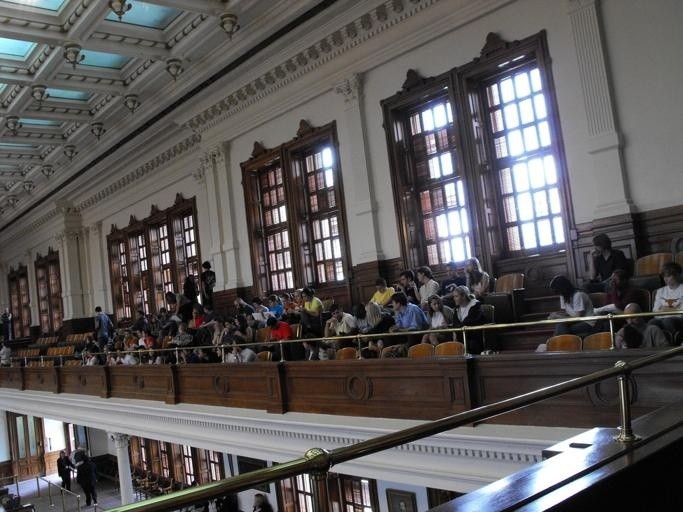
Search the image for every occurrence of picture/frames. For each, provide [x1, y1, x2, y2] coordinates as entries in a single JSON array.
[[384, 486, 418, 511]]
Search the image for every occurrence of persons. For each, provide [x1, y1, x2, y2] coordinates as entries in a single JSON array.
[[175, 480, 275, 512], [301, 257, 491, 362], [1, 336, 12, 366], [79, 262, 326, 367], [77, 455, 99, 506], [56, 450, 75, 495], [547, 233, 683, 350]]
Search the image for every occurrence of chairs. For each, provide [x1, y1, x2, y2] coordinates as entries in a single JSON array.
[[7, 250, 683, 369], [115, 465, 205, 511]]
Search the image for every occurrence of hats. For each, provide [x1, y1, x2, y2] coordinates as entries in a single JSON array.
[[202, 261, 211, 269]]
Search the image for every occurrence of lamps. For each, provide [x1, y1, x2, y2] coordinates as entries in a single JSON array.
[[165, 59, 184, 83], [64, 43, 85, 69], [90, 122, 106, 142], [42, 165, 54, 180], [219, 13, 240, 41], [124, 94, 141, 116], [6, 116, 22, 135], [64, 145, 78, 162], [109, 0, 132, 22], [23, 181, 35, 195], [32, 83, 50, 105], [7, 196, 17, 209]]
